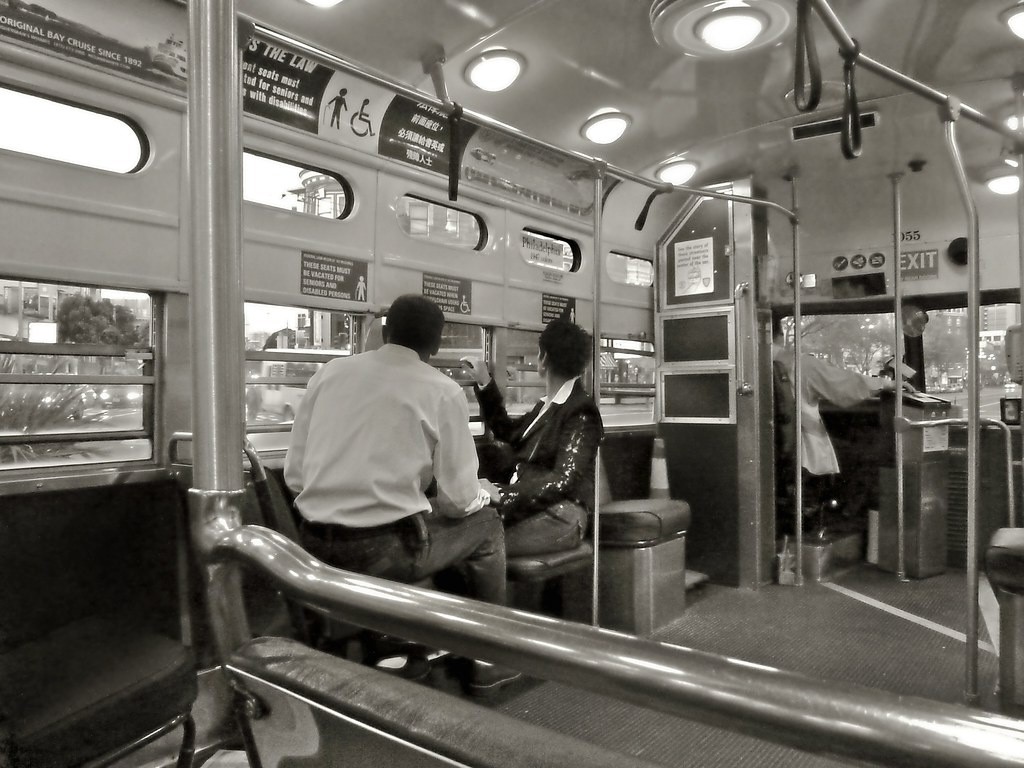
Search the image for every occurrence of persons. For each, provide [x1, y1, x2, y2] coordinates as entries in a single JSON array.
[[284, 295, 524, 698], [770, 309, 920, 533], [832, 275, 876, 298], [460, 319, 605, 558]]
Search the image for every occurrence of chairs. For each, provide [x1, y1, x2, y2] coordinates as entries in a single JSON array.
[[162, 426, 437, 651], [984, 526, 1024, 710], [464, 439, 597, 680], [587, 443, 694, 641], [769, 361, 856, 544], [1, 480, 200, 766]]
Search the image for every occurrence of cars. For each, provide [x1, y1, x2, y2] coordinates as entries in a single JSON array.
[[99, 383, 141, 409], [245, 383, 306, 422], [41, 384, 97, 411], [921, 382, 965, 393], [1005, 380, 1015, 389]]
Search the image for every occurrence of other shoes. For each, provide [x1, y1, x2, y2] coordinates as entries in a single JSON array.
[[825, 515, 870, 531]]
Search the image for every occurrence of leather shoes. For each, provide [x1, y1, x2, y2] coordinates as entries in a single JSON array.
[[467, 650, 526, 696], [367, 645, 431, 687]]
